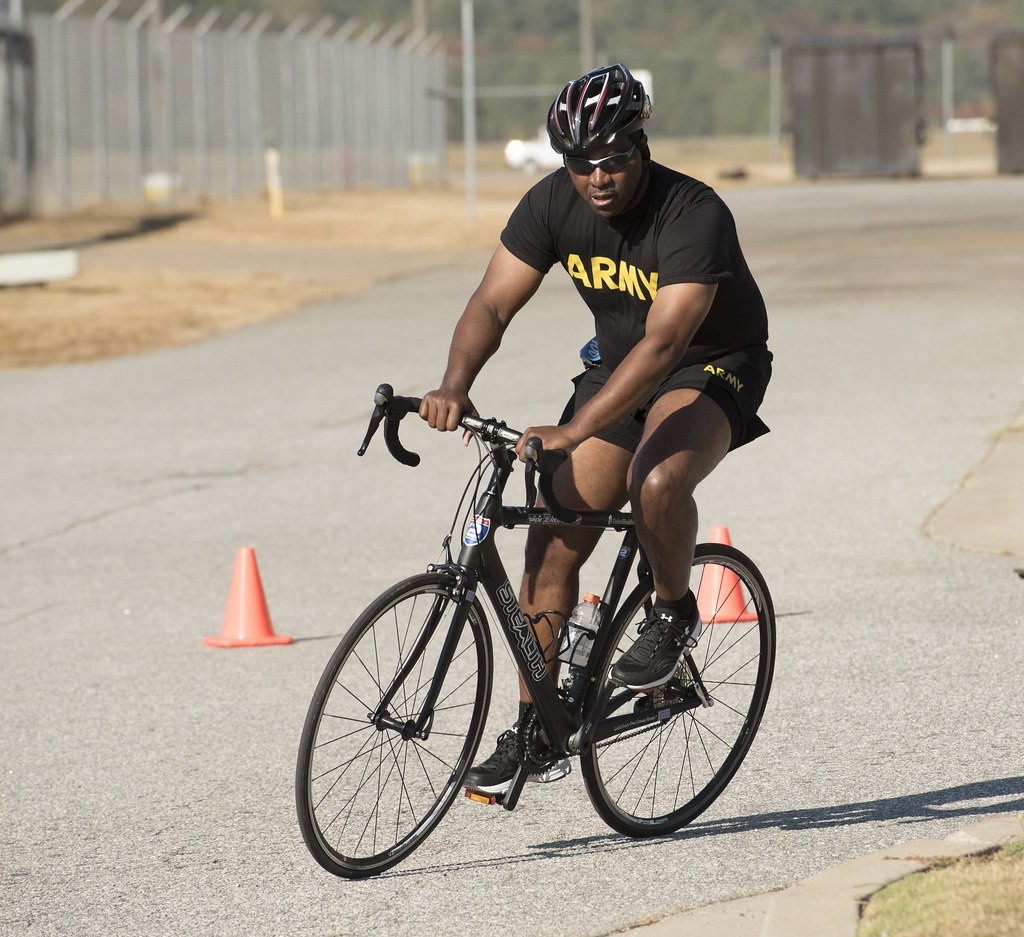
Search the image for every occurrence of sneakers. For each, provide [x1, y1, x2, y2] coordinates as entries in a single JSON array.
[[608, 590, 703, 689], [461, 721, 572, 795]]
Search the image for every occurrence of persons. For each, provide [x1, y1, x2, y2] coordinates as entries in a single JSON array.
[[419, 63, 773, 795]]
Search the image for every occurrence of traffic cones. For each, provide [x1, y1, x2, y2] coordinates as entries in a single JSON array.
[[690, 524, 762, 624], [201, 546, 294, 648]]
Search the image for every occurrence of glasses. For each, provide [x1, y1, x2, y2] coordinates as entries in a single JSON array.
[[562, 145, 639, 176]]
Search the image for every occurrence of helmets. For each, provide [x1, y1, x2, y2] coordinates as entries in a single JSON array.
[[547, 63, 655, 156]]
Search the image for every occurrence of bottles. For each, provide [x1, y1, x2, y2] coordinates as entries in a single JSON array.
[[557, 593, 600, 669]]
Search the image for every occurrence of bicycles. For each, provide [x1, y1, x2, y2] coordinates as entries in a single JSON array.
[[294, 388, 778, 880]]
